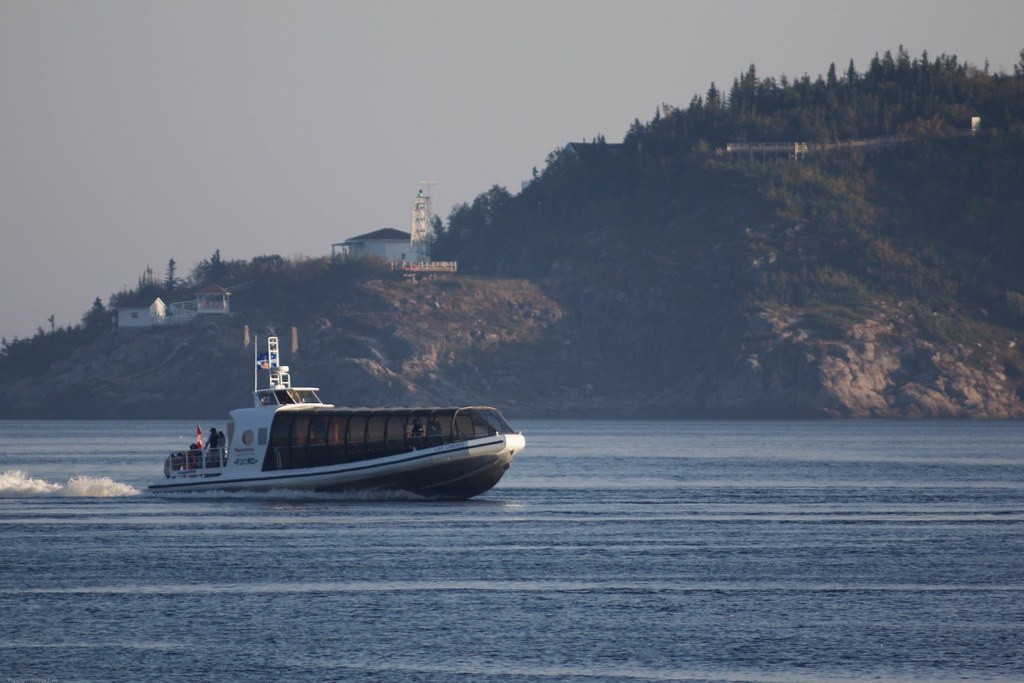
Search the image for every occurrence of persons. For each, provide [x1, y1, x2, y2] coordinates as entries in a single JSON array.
[[411, 418, 422, 436], [204, 427, 218, 467], [180, 444, 203, 471], [216, 431, 226, 466], [430, 416, 442, 435]]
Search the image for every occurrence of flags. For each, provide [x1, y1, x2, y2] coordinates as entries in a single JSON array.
[[196, 424, 202, 449]]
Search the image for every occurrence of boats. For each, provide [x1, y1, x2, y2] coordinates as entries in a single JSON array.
[[149, 333, 527, 502]]
